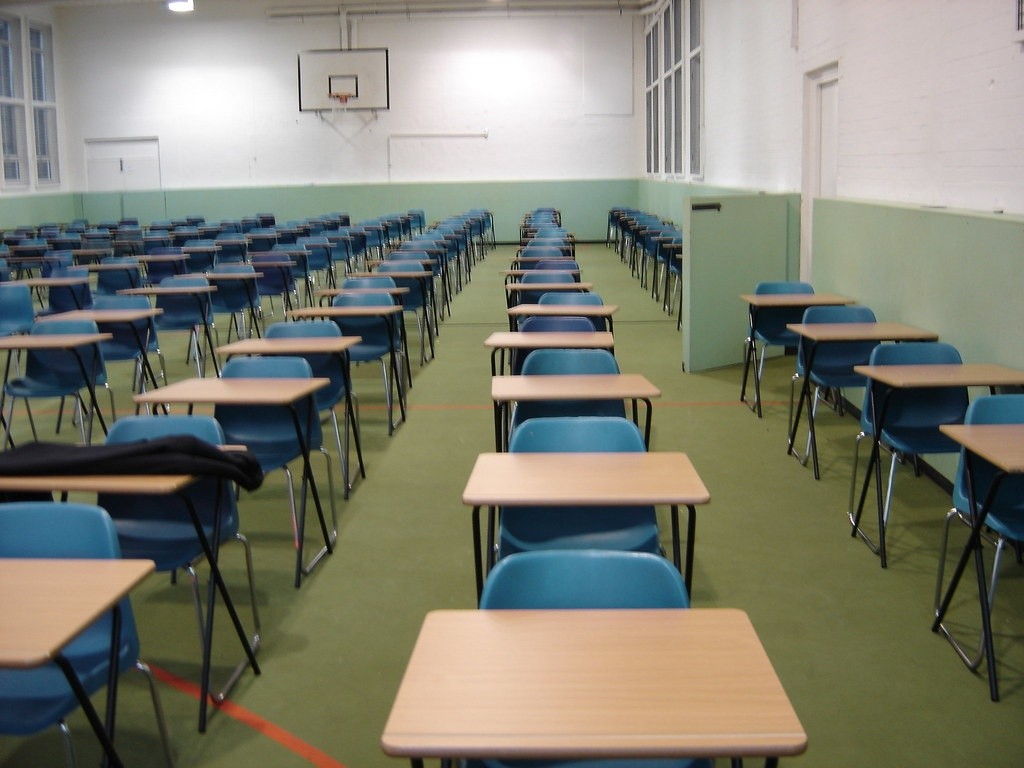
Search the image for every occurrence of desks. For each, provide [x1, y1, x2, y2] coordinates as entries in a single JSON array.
[[0, 242, 1024, 768]]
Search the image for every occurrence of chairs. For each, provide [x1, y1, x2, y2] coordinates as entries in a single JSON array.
[[931, 395, 1024, 672], [845, 339, 969, 555], [0, 318, 114, 447], [507, 349, 628, 444], [1, 501, 176, 768], [94, 413, 262, 705], [786, 304, 880, 467], [0, 204, 814, 412], [480, 550, 708, 768], [497, 414, 669, 575], [205, 356, 337, 575]]
